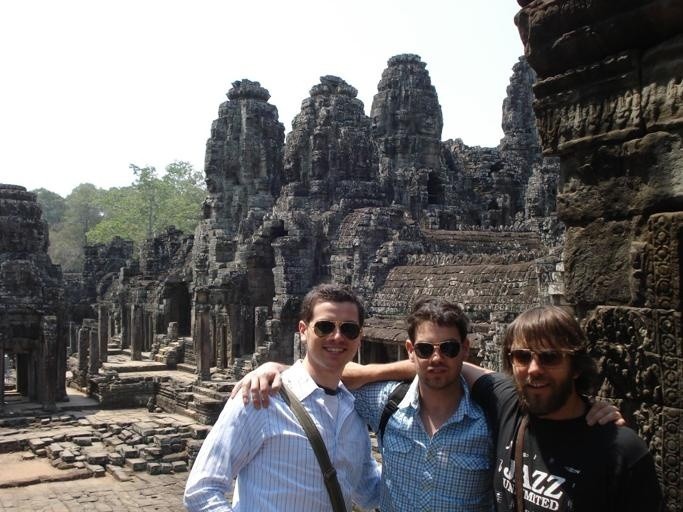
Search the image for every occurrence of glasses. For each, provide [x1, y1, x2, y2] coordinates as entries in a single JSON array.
[[308, 319, 363, 340], [506, 348, 575, 369], [411, 338, 462, 359]]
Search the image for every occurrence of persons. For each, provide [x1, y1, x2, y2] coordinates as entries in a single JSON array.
[[342, 307, 668, 512], [232, 299, 626, 512], [183, 283, 398, 512]]
